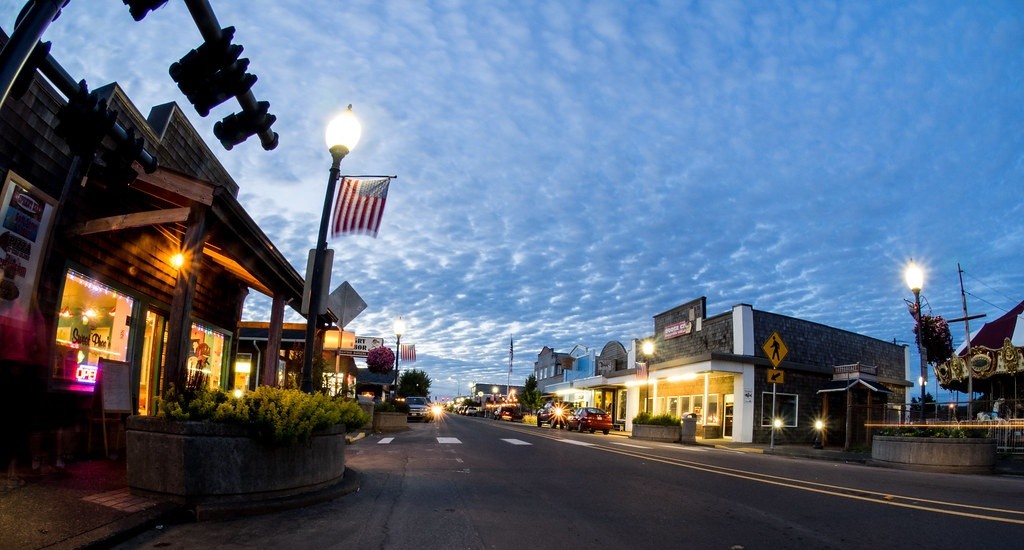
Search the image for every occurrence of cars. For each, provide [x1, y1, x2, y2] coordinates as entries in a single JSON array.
[[566, 407, 612, 435], [457, 406, 478, 416], [406, 397, 432, 422]]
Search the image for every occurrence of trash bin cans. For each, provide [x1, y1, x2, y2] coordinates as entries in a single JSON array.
[[357, 394, 375, 429]]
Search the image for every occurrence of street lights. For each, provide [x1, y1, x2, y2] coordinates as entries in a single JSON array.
[[297, 103, 362, 396], [642, 341, 654, 415], [394, 317, 405, 401], [906, 256, 930, 437]]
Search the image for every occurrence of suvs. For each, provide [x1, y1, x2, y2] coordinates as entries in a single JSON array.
[[537, 400, 575, 428]]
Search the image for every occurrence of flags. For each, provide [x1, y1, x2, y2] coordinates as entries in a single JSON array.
[[401, 344, 416, 362], [330, 177, 390, 239], [635, 362, 646, 380]]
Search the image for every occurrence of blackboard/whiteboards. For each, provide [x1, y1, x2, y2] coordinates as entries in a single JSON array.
[[100, 357, 133, 414], [681, 412, 698, 443]]
